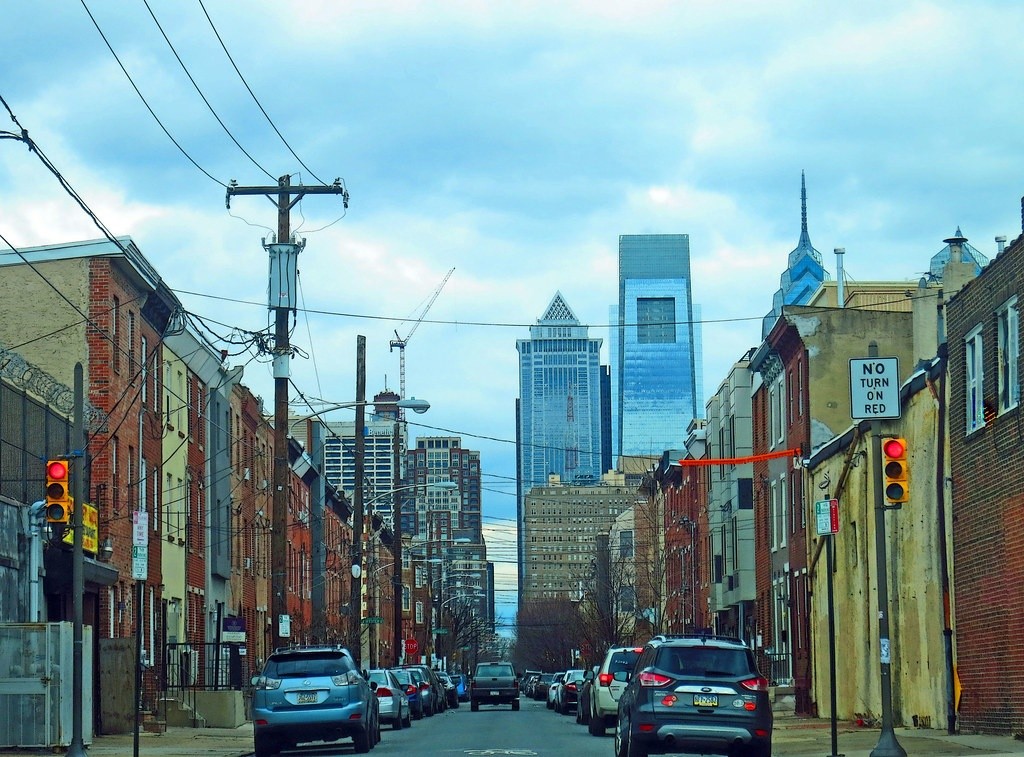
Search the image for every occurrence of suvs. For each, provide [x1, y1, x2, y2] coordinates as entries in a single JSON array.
[[251, 643, 382, 755], [582, 644, 685, 737], [470, 662, 520, 712], [613, 634, 775, 757]]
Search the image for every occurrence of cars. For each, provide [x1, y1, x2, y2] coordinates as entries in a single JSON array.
[[558, 669, 599, 715], [520, 669, 567, 711], [359, 669, 414, 731], [574, 665, 628, 724], [389, 669, 426, 720], [391, 665, 471, 717]]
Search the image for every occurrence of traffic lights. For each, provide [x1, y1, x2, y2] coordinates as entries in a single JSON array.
[[880, 437, 910, 503], [45, 459, 72, 525], [40, 547, 72, 608]]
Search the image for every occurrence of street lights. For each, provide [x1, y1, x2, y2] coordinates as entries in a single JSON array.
[[394, 538, 471, 665], [346, 482, 458, 667], [426, 571, 489, 668], [364, 557, 444, 671], [262, 396, 430, 652]]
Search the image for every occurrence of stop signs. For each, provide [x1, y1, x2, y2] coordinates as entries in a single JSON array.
[[404, 638, 419, 656]]
